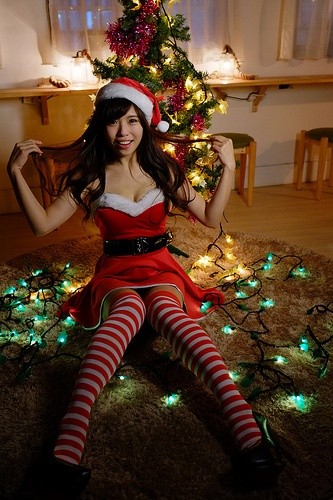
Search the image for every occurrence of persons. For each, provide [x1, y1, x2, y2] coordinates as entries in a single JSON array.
[[7, 77, 276, 492]]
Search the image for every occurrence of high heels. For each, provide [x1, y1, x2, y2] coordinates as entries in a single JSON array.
[[22, 444, 92, 497], [245, 411, 296, 481]]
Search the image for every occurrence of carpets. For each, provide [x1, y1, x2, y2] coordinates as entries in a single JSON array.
[[1, 226, 333, 500]]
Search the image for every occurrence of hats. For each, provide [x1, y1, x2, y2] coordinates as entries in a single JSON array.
[[95, 77, 170, 133]]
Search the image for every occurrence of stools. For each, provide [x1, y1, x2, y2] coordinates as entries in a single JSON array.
[[295, 128, 333, 201], [208, 133, 256, 207], [36, 142, 83, 231]]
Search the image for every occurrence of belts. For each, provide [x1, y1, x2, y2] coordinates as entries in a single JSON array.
[[103, 232, 173, 256]]
